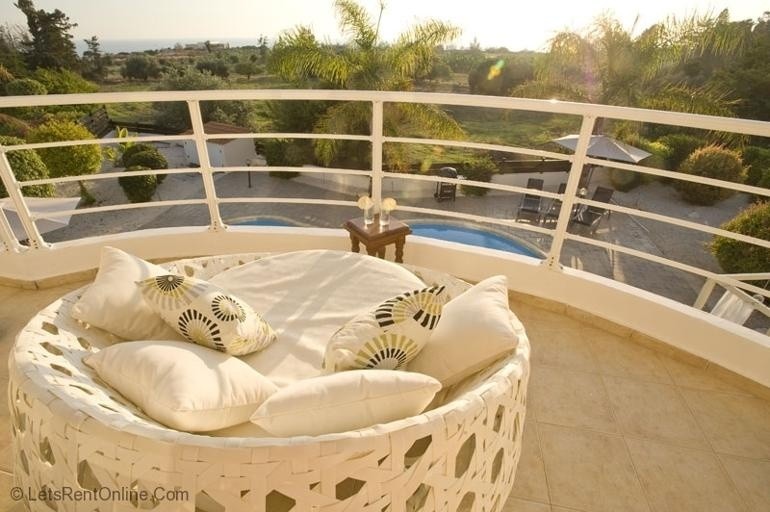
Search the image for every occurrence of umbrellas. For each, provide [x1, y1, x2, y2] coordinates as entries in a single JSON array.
[[551, 133, 654, 217]]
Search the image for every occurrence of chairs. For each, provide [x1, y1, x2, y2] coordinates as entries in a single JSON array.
[[571, 186, 615, 237], [543, 183, 567, 227], [3, 248, 532, 512], [515, 177, 544, 225]]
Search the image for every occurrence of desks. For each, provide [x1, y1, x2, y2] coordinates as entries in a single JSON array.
[[342, 213, 413, 264]]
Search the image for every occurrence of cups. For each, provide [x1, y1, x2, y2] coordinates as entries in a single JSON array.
[[362, 200, 375, 224], [379, 200, 392, 226]]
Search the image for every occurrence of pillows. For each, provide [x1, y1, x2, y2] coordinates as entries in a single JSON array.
[[322, 284, 447, 374], [404, 273, 520, 387], [133, 271, 277, 357], [249, 369, 443, 437], [81, 341, 269, 433], [70, 246, 181, 339]]
